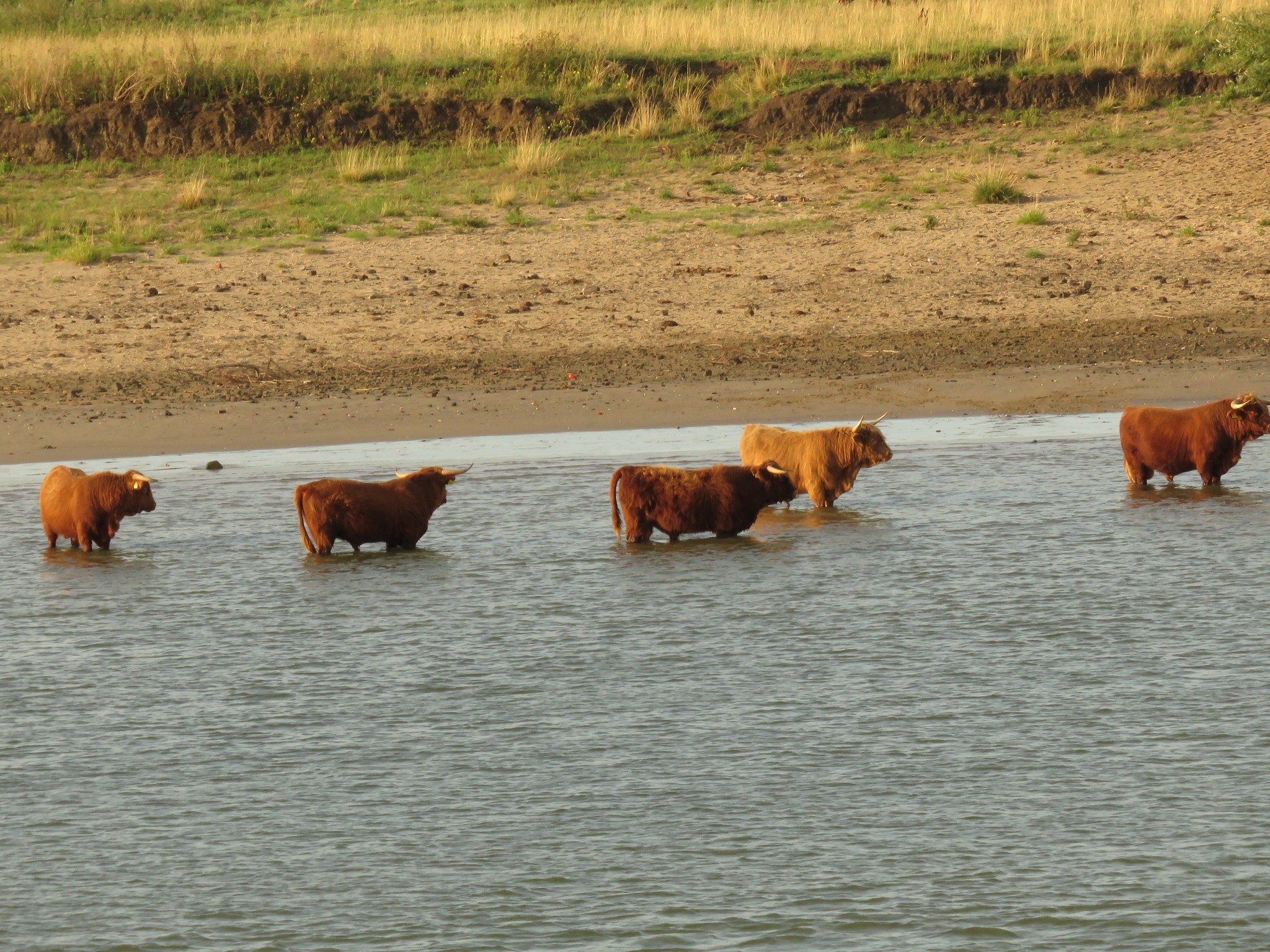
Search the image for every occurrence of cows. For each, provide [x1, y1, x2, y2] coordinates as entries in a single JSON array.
[[738, 411, 894, 509], [38, 464, 159, 553], [1117, 391, 1270, 489], [607, 458, 801, 546], [292, 462, 475, 555]]
